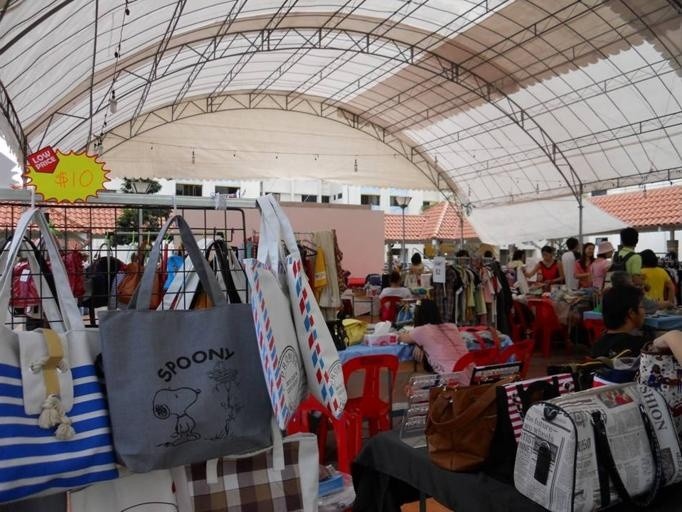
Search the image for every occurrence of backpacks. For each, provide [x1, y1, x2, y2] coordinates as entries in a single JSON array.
[[602, 250, 636, 289]]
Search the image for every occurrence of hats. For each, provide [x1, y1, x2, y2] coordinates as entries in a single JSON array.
[[597, 242, 614, 255], [456, 250, 468, 257]]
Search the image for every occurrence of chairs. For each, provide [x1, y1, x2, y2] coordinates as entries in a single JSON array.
[[287, 353, 401, 511]]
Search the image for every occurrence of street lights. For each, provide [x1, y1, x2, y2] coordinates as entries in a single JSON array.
[[394, 196, 412, 287]]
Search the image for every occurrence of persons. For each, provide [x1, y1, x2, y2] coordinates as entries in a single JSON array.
[[484, 250, 494, 257], [455, 249, 468, 256], [408, 253, 425, 273], [588, 283, 654, 356], [563, 236, 582, 289], [603, 226, 652, 297], [639, 248, 675, 301], [572, 242, 595, 291], [521, 246, 565, 286], [611, 269, 674, 314], [591, 241, 612, 291], [387, 270, 403, 288]]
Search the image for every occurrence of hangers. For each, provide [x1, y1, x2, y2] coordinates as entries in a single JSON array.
[[445, 255, 496, 271], [251, 229, 322, 257]]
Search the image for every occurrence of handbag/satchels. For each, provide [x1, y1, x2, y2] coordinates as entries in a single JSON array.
[[493, 373, 575, 467], [99, 302, 273, 472], [171, 434, 319, 512], [11, 253, 215, 310], [593, 367, 634, 386], [343, 317, 367, 344], [517, 383, 682, 512], [0, 324, 117, 505], [640, 348, 682, 433], [425, 371, 522, 471], [66, 467, 178, 512], [546, 356, 617, 392], [242, 251, 348, 432], [453, 326, 534, 380]]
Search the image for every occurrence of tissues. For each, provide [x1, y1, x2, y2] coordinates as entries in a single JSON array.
[[362, 320, 398, 347]]
